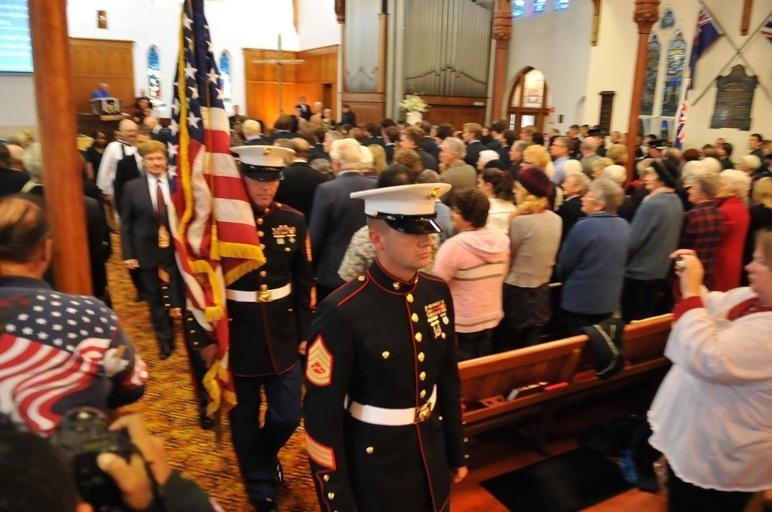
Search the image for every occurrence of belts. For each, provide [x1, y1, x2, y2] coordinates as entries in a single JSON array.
[[224, 283, 293, 306], [348, 386, 444, 427]]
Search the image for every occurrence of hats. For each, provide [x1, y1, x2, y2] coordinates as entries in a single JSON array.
[[519, 167, 555, 198], [348, 182, 454, 235], [228, 143, 297, 180], [651, 158, 680, 189]]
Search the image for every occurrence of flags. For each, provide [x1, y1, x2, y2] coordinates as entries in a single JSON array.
[[685, 7, 728, 96], [758, 12, 772, 47], [166, 0, 268, 425]]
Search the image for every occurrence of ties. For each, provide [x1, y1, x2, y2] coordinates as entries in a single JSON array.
[[155, 179, 166, 216]]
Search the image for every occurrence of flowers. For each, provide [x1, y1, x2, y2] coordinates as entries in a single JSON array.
[[394, 89, 434, 112]]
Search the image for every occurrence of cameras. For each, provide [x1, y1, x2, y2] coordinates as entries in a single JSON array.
[[47, 407, 134, 503], [672, 257, 686, 272]]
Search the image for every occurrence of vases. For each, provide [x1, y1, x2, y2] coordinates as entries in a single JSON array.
[[406, 111, 423, 127]]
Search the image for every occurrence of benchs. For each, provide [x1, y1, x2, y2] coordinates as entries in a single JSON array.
[[452, 313, 678, 493]]
